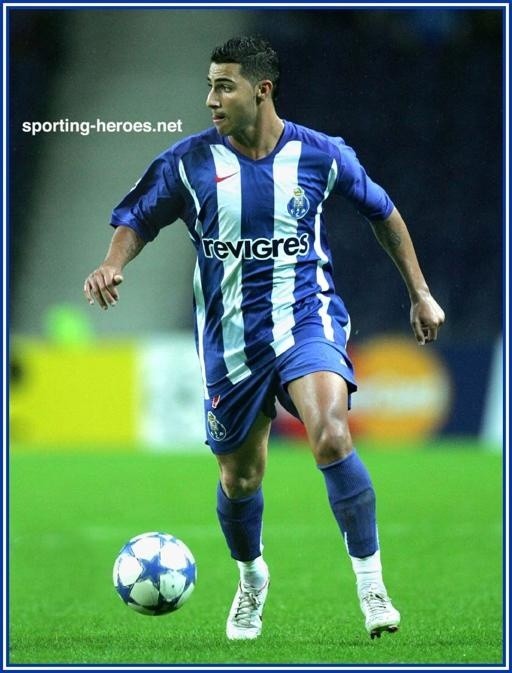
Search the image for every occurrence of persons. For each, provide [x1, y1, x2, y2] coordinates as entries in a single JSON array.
[[84, 37, 446, 641]]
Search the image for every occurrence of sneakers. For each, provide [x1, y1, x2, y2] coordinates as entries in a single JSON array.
[[357, 580, 401, 638], [226, 577, 269, 642]]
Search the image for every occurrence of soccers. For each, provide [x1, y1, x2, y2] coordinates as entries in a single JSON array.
[[113, 533, 197, 615]]
